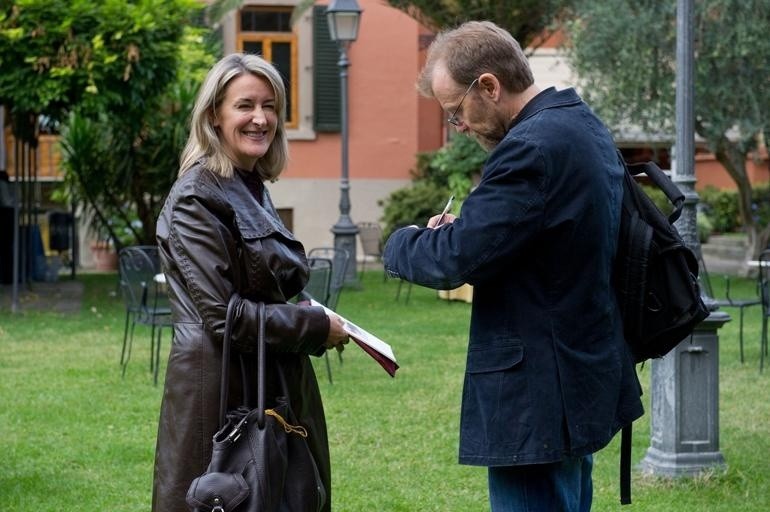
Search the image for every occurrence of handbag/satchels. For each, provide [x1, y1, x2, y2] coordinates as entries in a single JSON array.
[[187, 294, 328, 512]]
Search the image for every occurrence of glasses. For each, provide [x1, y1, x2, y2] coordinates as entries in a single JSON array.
[[447, 79, 479, 128]]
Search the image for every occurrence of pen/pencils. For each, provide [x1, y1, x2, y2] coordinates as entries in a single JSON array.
[[433, 196, 457, 228]]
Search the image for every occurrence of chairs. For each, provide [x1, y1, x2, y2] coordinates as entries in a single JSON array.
[[681, 241, 770, 373], [358, 221, 443, 308], [115, 242, 175, 388], [285, 244, 350, 386]]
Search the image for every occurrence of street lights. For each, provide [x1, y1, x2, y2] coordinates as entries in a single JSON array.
[[322, 0, 365, 292]]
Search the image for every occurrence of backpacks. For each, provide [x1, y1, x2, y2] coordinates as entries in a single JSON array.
[[602, 113, 711, 505]]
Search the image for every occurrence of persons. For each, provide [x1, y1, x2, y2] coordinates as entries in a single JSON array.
[[152, 54, 349, 512], [383, 20, 644, 512]]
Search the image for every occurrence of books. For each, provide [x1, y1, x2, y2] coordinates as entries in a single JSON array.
[[310, 298, 400, 378]]
